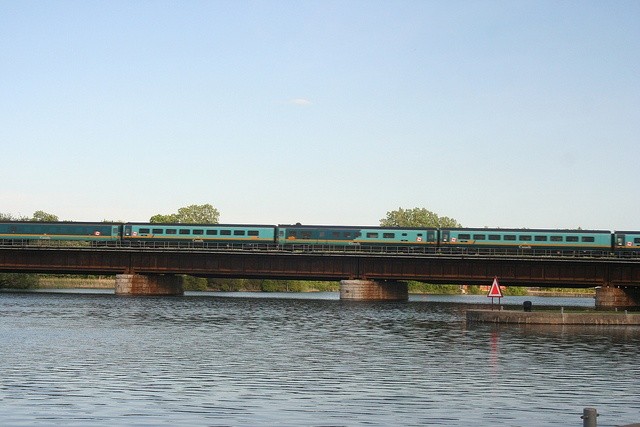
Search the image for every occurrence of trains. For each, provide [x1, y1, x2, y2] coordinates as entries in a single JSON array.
[[0, 219, 640, 257]]
